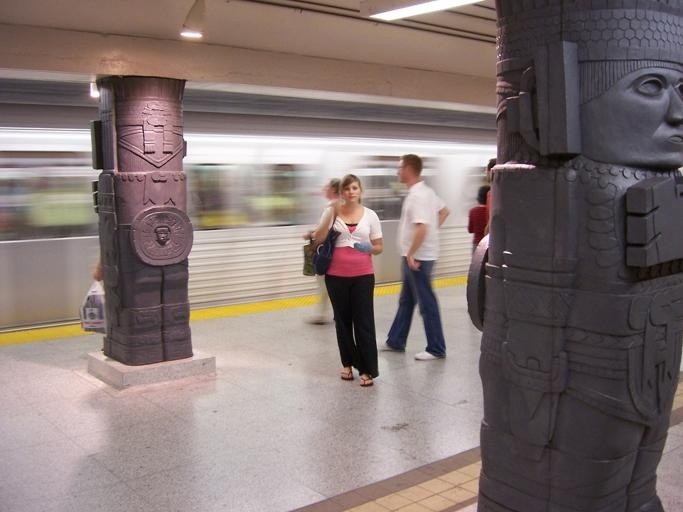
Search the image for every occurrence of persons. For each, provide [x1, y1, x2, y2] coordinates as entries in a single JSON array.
[[566, 7, 683, 171], [311, 175, 384, 387], [300, 178, 341, 327], [376, 154, 451, 360], [467, 184, 490, 257], [482, 157, 498, 238], [156, 227, 170, 245], [93, 257, 104, 282]]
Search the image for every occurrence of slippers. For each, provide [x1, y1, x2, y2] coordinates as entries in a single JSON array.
[[360, 377, 373, 387], [340, 369, 353, 381]]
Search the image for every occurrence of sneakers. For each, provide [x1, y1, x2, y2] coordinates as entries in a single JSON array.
[[377, 343, 404, 351], [415, 351, 445, 360]]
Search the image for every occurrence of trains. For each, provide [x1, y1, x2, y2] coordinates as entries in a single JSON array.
[[1, 123, 504, 337]]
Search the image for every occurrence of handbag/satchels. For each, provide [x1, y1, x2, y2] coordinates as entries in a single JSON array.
[[314, 227, 341, 275], [303, 238, 315, 276]]
